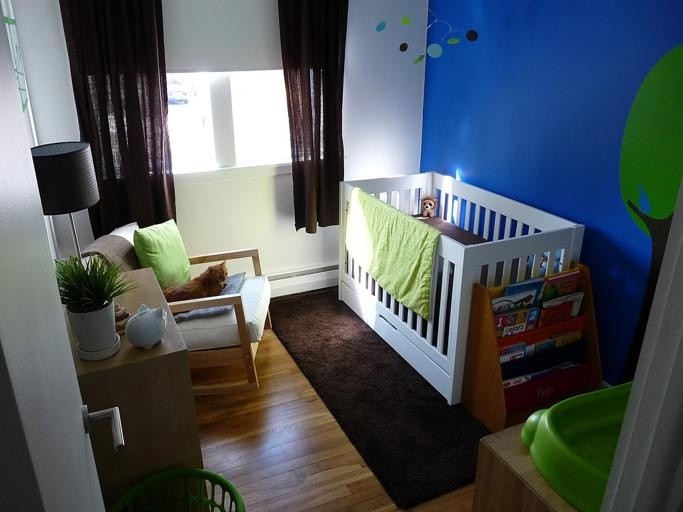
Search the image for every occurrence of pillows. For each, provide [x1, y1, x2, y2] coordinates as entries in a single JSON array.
[[134, 220, 191, 291]]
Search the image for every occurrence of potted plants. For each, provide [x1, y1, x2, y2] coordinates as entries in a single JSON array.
[[54, 250, 140, 361]]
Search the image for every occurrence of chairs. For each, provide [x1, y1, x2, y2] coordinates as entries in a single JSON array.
[[81, 222, 272, 397]]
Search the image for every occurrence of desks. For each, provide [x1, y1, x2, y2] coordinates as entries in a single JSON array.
[[472, 422, 578, 512], [62, 267, 210, 512]]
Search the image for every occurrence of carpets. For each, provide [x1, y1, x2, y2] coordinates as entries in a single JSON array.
[[268, 284, 493, 512]]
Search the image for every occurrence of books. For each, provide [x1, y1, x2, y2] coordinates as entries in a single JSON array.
[[491, 268, 589, 388]]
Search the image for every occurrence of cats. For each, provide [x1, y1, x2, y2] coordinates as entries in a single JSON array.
[[163, 260, 229, 316]]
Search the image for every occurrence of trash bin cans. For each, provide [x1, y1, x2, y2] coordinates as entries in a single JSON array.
[[113, 467, 244, 511]]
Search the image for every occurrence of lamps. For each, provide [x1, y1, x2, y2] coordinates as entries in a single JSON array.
[[30, 141, 100, 264]]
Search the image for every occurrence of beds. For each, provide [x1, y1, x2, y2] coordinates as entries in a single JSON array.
[[338, 171, 585, 405]]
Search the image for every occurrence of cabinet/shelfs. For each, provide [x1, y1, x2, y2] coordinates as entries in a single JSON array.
[[460, 260, 602, 434]]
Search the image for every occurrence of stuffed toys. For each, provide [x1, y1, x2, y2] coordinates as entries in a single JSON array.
[[422, 197, 437, 218]]
